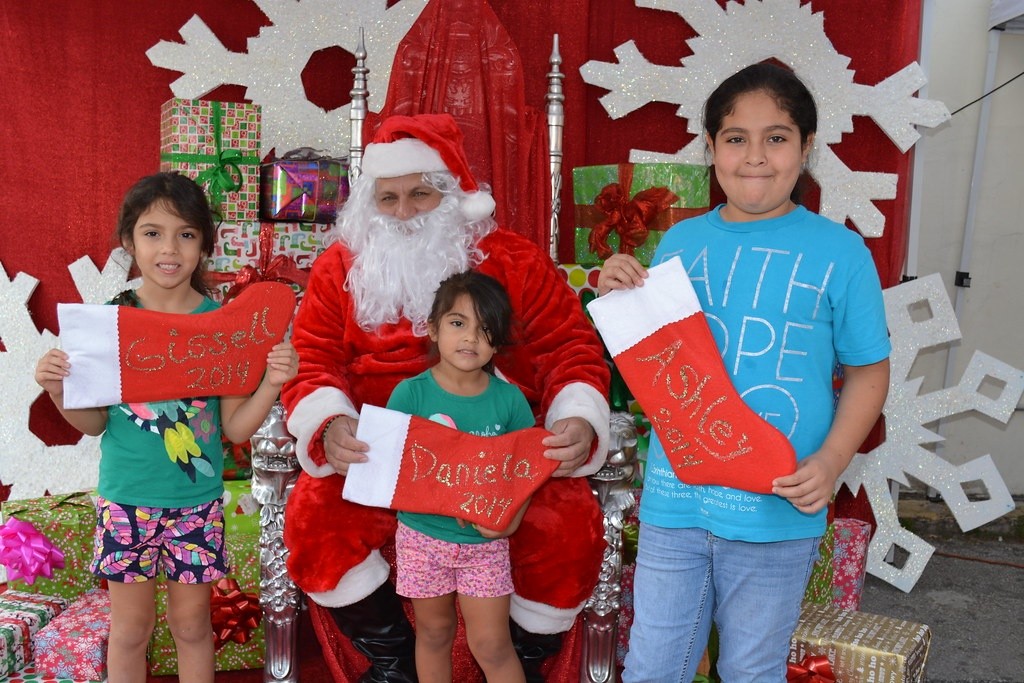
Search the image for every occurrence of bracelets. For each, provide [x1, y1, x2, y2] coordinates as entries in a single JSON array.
[[323, 416, 341, 439]]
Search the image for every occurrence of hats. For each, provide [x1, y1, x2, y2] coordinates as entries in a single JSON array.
[[361, 115, 495, 221]]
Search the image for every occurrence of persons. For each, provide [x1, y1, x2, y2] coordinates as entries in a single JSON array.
[[35, 176, 300, 683], [384, 270, 535, 683], [597, 63, 889, 683], [280, 116, 610, 683]]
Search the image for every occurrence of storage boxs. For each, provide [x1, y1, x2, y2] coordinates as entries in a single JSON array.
[[200, 222, 336, 341], [223, 479, 262, 534], [159, 99, 263, 222], [804, 523, 836, 604], [1, 491, 98, 602], [32, 590, 111, 681], [833, 517, 872, 611], [571, 163, 710, 268], [557, 263, 603, 317], [259, 159, 348, 224], [788, 600, 932, 683], [148, 530, 265, 677], [0, 588, 71, 683]]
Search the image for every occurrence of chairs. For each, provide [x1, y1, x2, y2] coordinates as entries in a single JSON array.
[[248, 0, 642, 683]]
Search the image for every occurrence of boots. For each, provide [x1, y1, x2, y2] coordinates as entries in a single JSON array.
[[484, 592, 586, 683], [308, 548, 419, 683]]
